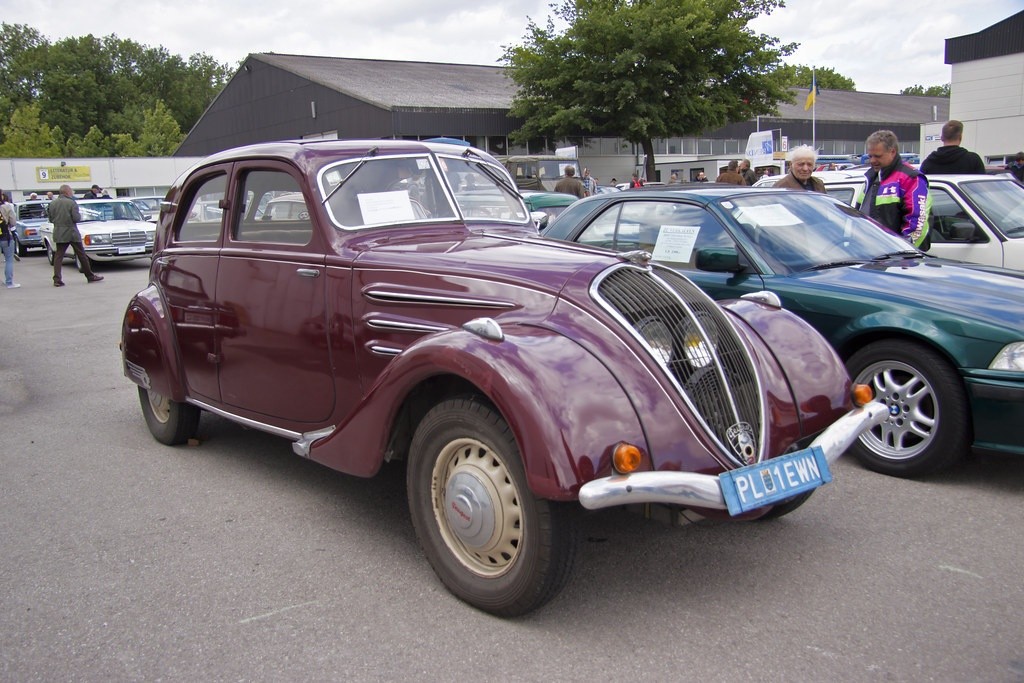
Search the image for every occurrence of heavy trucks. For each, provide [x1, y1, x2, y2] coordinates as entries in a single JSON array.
[[493, 156, 586, 194]]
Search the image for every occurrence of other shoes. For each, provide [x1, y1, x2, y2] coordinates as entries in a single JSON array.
[[6, 283, 20, 289], [88, 274, 104, 282], [54, 280, 65, 287], [14, 254, 21, 261]]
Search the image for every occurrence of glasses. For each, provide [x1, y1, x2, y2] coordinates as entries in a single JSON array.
[[868, 154, 883, 158]]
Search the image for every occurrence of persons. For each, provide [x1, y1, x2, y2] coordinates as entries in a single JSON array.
[[554, 154, 916, 196], [772, 144, 827, 193], [920, 120, 988, 175], [0, 185, 112, 288], [49, 185, 104, 287], [855, 130, 932, 253], [1005, 152, 1024, 185]]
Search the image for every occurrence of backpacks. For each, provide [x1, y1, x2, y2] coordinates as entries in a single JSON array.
[[0, 211, 10, 239], [633, 179, 642, 188]]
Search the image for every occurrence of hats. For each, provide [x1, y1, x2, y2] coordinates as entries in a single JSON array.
[[92, 185, 103, 190], [564, 164, 575, 174]]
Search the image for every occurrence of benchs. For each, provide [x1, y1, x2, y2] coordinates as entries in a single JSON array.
[[181, 219, 312, 243]]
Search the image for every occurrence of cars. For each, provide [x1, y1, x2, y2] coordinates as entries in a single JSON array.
[[819, 174, 1024, 273], [540, 187, 1024, 478], [118, 137, 889, 618], [38, 198, 157, 273], [261, 192, 310, 219], [128, 195, 167, 222], [12, 200, 51, 258]]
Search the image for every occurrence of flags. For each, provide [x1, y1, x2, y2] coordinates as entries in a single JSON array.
[[805, 71, 820, 111]]
